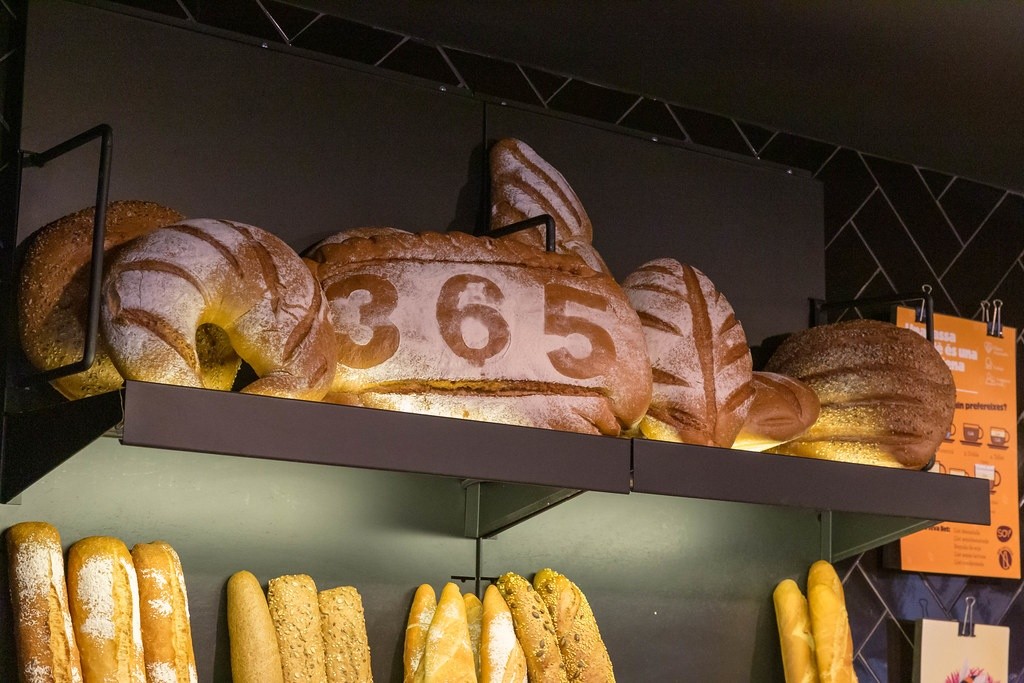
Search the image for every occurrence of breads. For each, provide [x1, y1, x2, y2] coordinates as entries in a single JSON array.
[[226, 570, 375, 683], [403, 568, 617, 683], [16, 132, 958, 471], [4, 520, 197, 683], [773, 559, 860, 682]]
